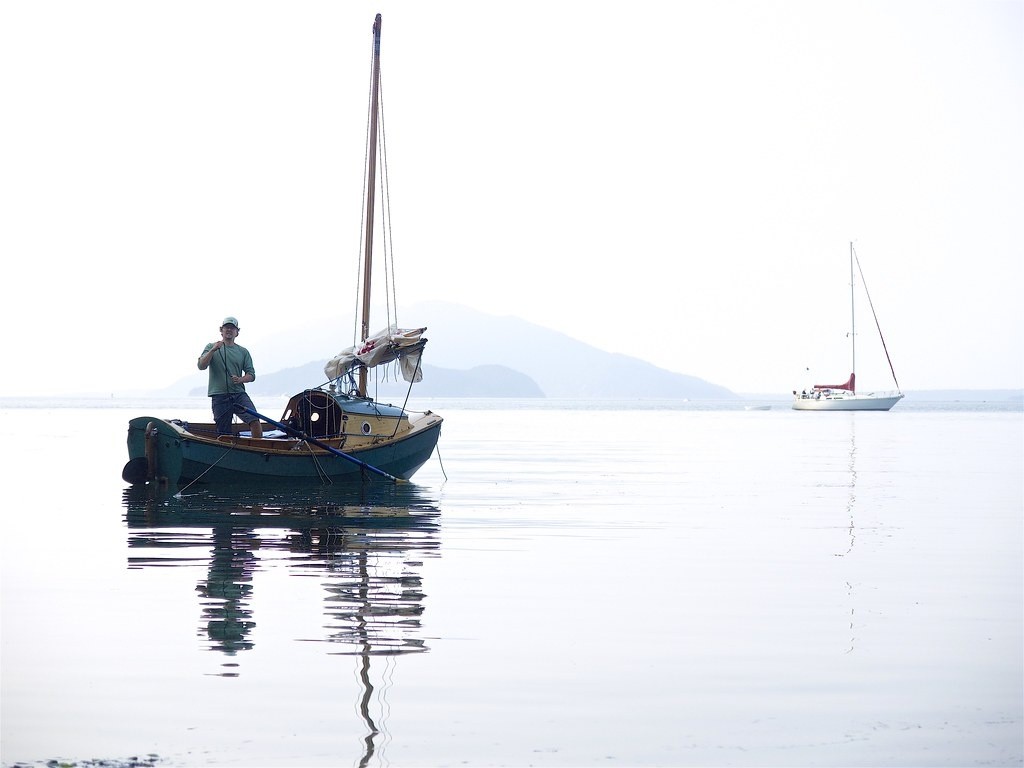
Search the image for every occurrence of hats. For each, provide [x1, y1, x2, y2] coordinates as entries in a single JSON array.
[[222, 317, 238, 329]]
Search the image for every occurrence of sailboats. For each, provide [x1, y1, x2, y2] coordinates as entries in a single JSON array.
[[122, 12, 465, 490], [790, 242, 904, 412]]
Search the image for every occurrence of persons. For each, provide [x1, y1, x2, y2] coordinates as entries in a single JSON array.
[[197, 316, 263, 438]]
